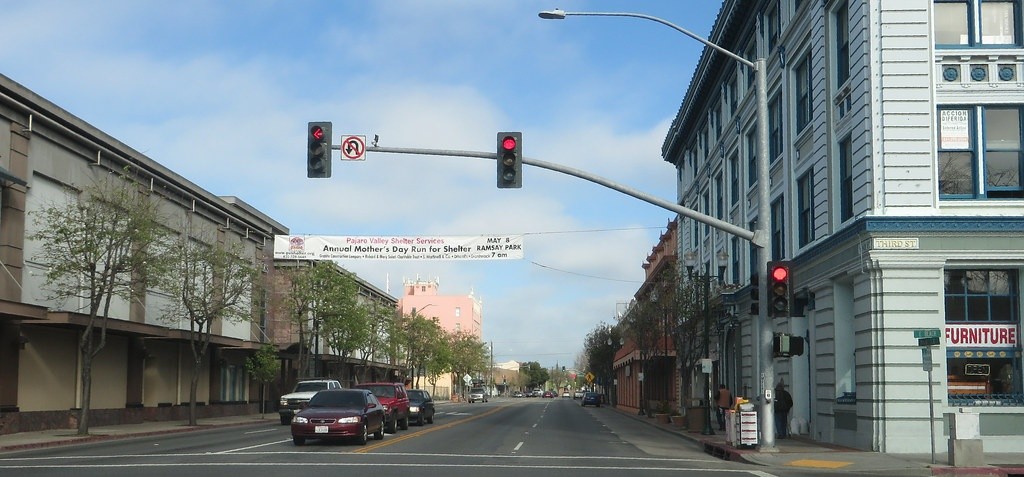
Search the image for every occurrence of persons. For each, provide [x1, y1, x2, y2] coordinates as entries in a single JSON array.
[[774, 383, 794, 439], [714, 384, 733, 432]]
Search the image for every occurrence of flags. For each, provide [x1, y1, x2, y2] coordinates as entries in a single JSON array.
[[0, 299, 51, 322], [13, 308, 170, 337]]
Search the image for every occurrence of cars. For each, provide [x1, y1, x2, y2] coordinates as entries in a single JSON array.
[[562, 391, 570, 398], [290, 388, 386, 444], [581, 392, 602, 408], [405, 389, 435, 426], [574, 391, 584, 399], [512, 388, 558, 398]]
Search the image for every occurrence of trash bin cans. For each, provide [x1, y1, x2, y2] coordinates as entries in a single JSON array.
[[685, 398, 706, 433]]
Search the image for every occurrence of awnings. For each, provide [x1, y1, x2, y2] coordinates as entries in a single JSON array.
[[144, 328, 243, 347], [316, 354, 407, 374], [221, 337, 278, 355], [272, 339, 312, 357]]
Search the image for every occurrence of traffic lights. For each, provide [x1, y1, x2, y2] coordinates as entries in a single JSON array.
[[307, 121, 332, 179], [496, 131, 522, 189], [751, 272, 759, 316], [795, 287, 816, 310], [767, 260, 794, 318], [503, 378, 506, 385], [562, 366, 566, 372], [775, 335, 789, 353]]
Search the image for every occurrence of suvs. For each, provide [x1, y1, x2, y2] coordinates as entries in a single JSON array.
[[354, 382, 410, 434], [278, 378, 343, 425], [467, 388, 489, 403]]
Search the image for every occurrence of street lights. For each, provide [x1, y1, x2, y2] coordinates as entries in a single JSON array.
[[537, 9, 777, 446], [411, 302, 438, 389]]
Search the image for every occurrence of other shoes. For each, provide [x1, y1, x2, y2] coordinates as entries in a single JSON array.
[[778, 435, 787, 439], [718, 426, 724, 431]]
